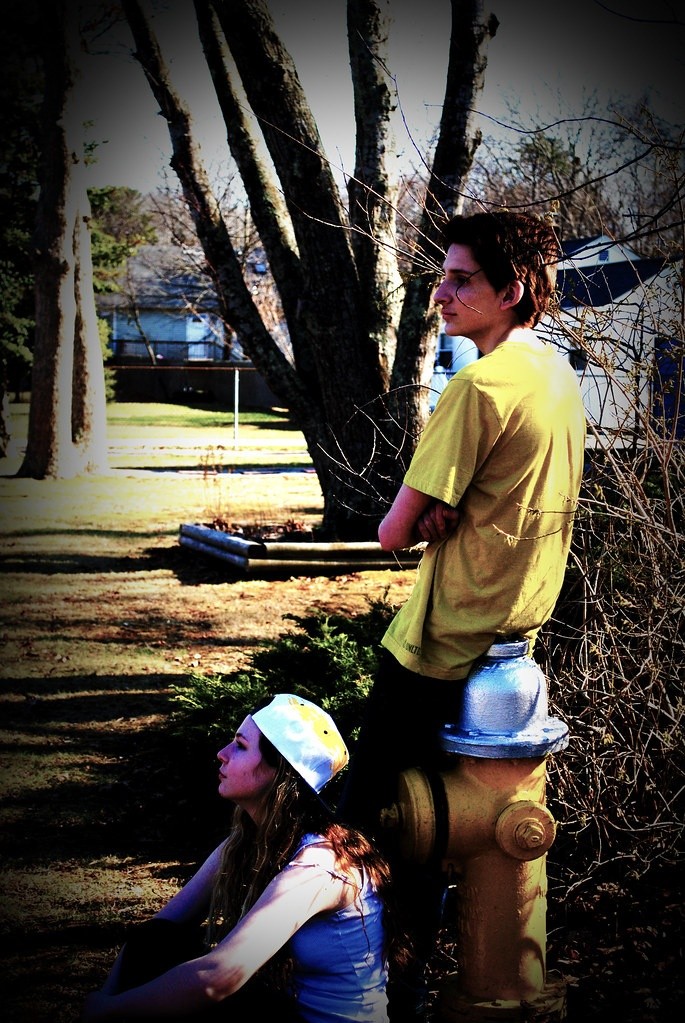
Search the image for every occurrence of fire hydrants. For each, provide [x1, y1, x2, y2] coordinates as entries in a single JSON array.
[[391, 635, 572, 1023]]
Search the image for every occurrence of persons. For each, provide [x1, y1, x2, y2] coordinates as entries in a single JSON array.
[[80, 693, 415, 1023], [333, 209, 586, 835]]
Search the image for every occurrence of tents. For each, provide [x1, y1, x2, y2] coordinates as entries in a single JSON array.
[[527, 232, 685, 431]]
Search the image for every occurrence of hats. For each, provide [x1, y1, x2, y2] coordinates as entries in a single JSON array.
[[251, 693, 351, 814]]
